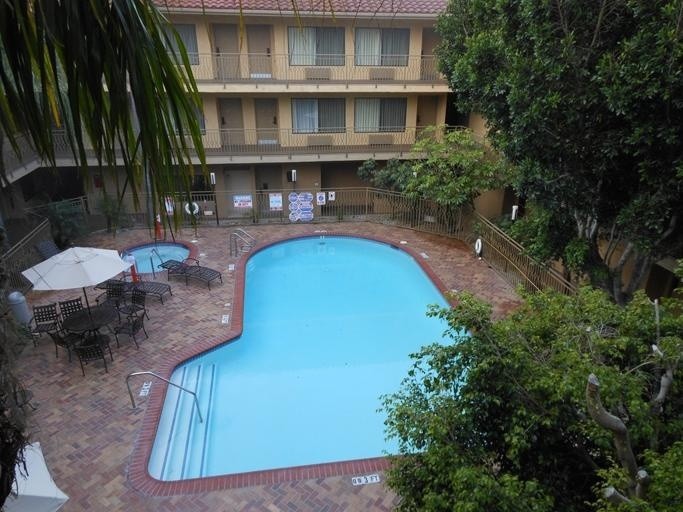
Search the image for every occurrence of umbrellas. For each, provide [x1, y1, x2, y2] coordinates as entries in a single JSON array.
[[0, 441, 70, 512], [20, 245, 134, 315]]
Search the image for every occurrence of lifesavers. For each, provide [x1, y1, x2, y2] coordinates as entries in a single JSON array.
[[185, 202, 199, 216], [475, 238, 482, 254]]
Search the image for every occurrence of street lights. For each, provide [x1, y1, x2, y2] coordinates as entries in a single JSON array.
[[291, 170, 297, 191], [510, 205, 518, 225], [209, 172, 220, 225]]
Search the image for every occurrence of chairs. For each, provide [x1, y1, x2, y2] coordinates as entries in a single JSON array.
[[115, 311, 148, 348], [94, 279, 173, 305], [117, 288, 149, 325], [96, 279, 126, 309], [28, 302, 62, 346], [73, 339, 114, 376], [158, 258, 223, 291], [46, 331, 85, 362], [55, 296, 83, 333]]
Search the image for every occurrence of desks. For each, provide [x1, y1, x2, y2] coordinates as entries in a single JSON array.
[[62, 306, 114, 340]]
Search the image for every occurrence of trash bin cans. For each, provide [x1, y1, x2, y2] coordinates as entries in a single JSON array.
[[7, 291, 32, 324]]
